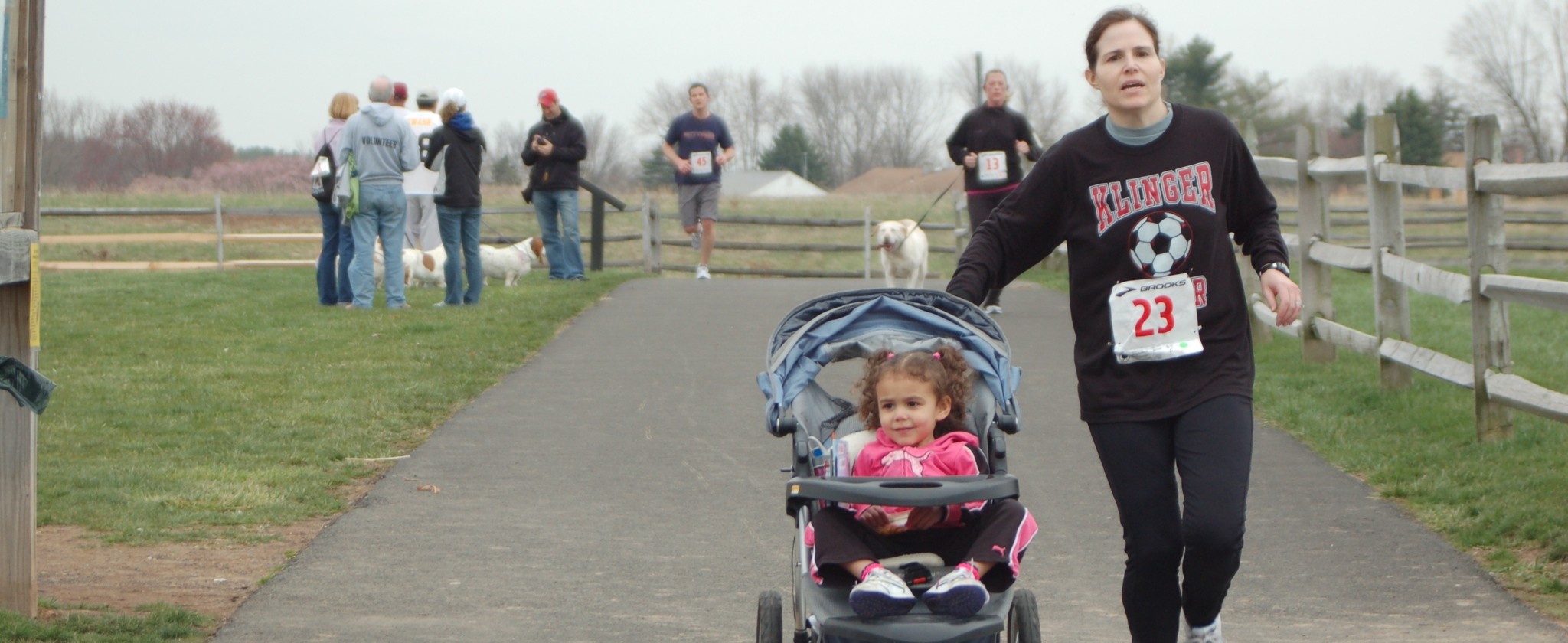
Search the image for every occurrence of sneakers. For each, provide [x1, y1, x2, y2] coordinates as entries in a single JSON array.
[[921, 566, 991, 615], [849, 568, 917, 617]]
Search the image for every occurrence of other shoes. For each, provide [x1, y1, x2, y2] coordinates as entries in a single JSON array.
[[691, 221, 703, 250], [696, 264, 711, 280], [1182, 613, 1223, 643], [573, 276, 589, 281], [433, 301, 447, 307], [346, 305, 357, 309], [336, 302, 353, 307], [401, 304, 412, 309], [984, 305, 1003, 314], [549, 275, 565, 280]]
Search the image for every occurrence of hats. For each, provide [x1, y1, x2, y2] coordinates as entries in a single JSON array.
[[393, 82, 408, 99], [416, 90, 439, 101], [537, 88, 557, 107], [442, 88, 467, 107]]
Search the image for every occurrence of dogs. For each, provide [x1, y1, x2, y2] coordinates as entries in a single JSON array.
[[871, 219, 930, 290], [337, 235, 447, 289], [477, 236, 546, 286]]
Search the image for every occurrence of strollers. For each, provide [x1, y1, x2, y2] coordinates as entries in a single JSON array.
[[756, 286, 1044, 643]]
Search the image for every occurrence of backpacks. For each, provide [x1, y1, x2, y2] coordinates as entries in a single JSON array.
[[310, 127, 343, 203]]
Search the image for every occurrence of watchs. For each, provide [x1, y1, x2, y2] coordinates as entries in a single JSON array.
[[1258, 261, 1291, 278]]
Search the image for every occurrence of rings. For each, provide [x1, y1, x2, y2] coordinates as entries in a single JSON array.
[[1297, 303, 1302, 308]]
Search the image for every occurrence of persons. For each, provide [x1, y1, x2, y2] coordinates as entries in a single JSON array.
[[310, 76, 487, 307], [810, 345, 1040, 618], [661, 83, 737, 280], [520, 89, 589, 281], [947, 70, 1045, 313], [946, 7, 1304, 643]]
